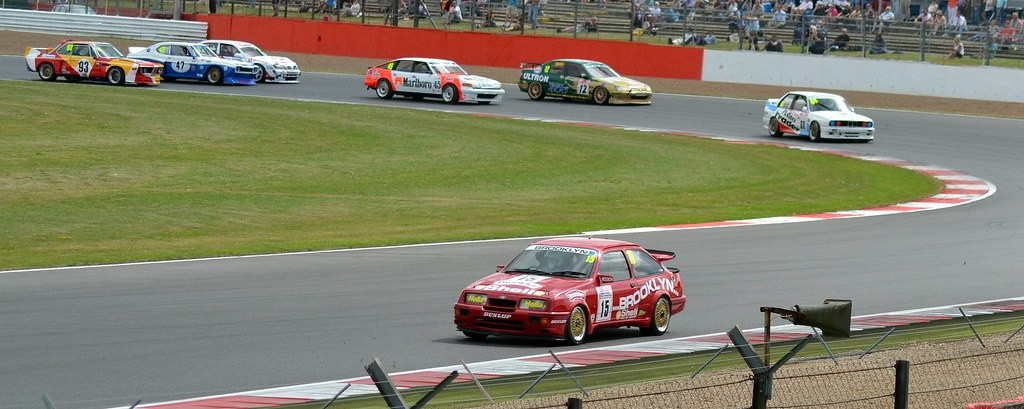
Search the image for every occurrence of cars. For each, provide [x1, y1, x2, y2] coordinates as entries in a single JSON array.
[[364, 57, 505, 105], [125, 42, 258, 86], [761, 92, 874, 142], [454, 235, 687, 346], [197, 38, 301, 84], [25, 40, 164, 87], [518, 58, 653, 106]]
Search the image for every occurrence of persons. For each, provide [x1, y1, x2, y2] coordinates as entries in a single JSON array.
[[192, 0, 543, 32], [556, 17, 598, 33], [629, 0, 967, 59]]
